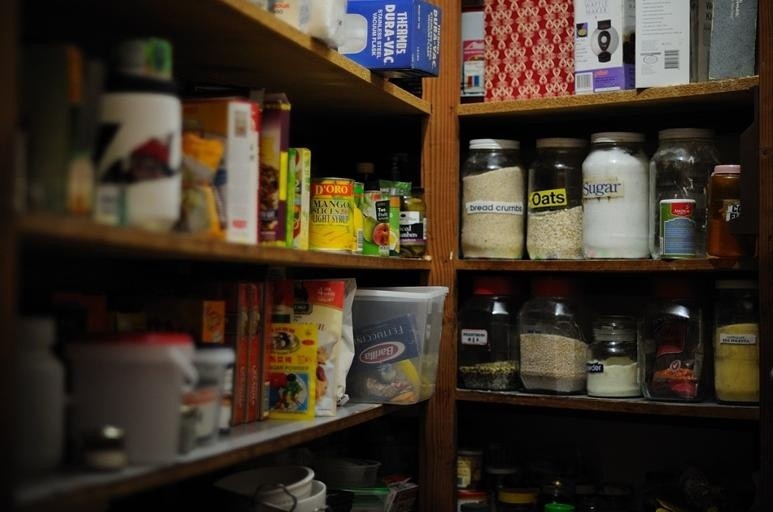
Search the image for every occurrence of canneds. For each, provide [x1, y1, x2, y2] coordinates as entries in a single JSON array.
[[308, 177, 354, 254], [364, 191, 390, 257], [456, 452, 576, 512], [353, 182, 364, 255], [399, 196, 426, 258], [389, 195, 401, 257]]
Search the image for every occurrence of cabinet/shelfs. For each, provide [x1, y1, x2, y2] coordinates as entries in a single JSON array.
[[443, 1, 772, 510], [0, 1, 442, 512]]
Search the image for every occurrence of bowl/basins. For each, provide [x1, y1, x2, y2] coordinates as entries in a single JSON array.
[[279, 480, 328, 511], [214, 464, 316, 504]]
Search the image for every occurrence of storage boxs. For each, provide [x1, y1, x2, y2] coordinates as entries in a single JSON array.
[[348, 285, 449, 404]]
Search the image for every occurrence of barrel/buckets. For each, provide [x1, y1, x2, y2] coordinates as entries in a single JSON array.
[[71, 334, 199, 468], [217, 464, 329, 512]]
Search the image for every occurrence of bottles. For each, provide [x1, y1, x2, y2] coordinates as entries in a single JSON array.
[[457, 279, 519, 391], [643, 279, 703, 402], [456, 447, 538, 512], [711, 279, 760, 405], [517, 278, 585, 394], [459, 126, 746, 259], [588, 315, 641, 401]]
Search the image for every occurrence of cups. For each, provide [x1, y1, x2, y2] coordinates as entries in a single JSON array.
[[541, 476, 577, 512]]
